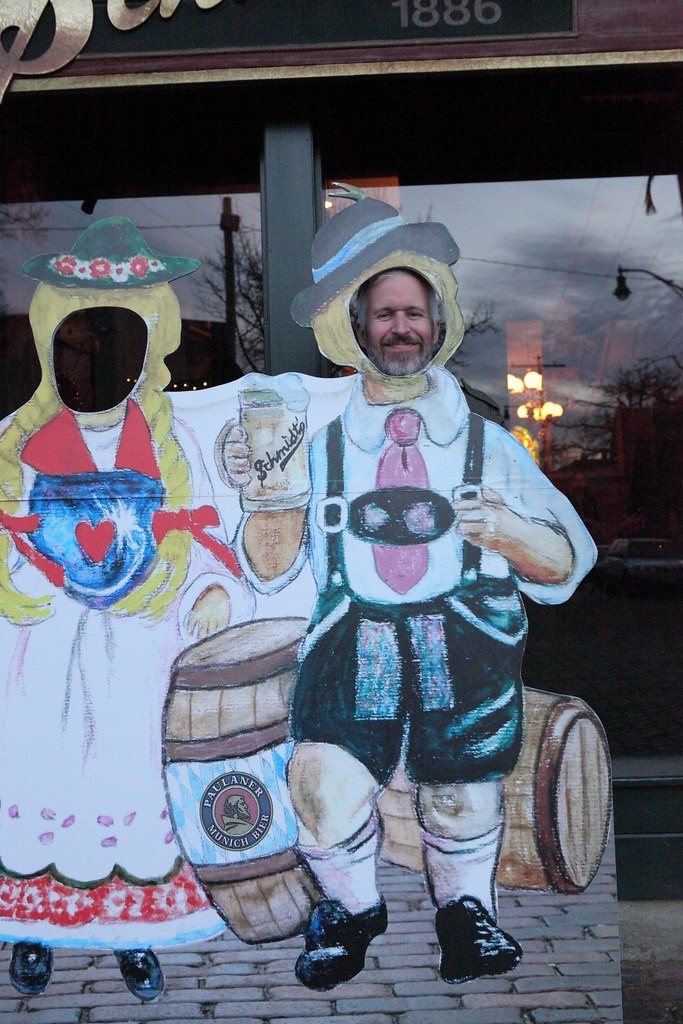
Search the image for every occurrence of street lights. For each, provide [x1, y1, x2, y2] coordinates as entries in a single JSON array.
[[220, 196, 242, 382]]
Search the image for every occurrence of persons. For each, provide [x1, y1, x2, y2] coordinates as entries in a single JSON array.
[[349, 267, 444, 376]]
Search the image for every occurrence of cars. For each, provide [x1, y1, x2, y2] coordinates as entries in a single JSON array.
[[591, 545, 613, 573]]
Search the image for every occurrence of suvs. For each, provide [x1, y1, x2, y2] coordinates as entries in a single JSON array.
[[604, 538, 683, 599]]
